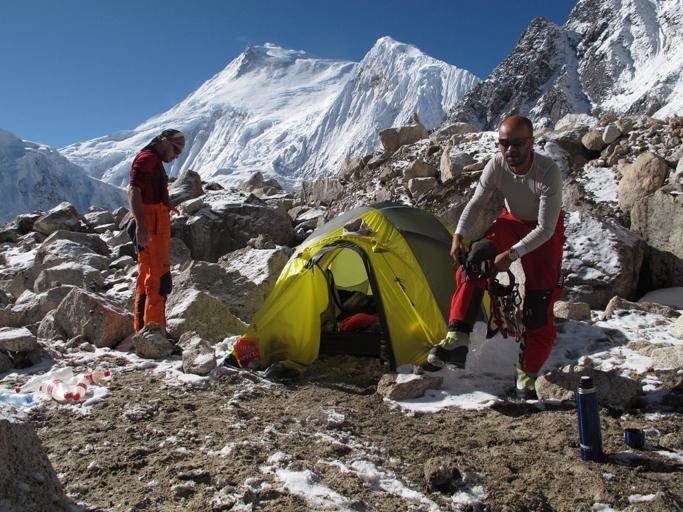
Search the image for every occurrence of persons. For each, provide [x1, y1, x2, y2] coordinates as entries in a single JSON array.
[[128, 129, 185, 355], [424, 113, 566, 401]]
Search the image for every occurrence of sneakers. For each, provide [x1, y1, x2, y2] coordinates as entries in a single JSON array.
[[516, 381, 540, 401], [428, 342, 468, 370]]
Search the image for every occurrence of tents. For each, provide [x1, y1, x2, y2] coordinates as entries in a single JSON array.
[[222, 198, 506, 379]]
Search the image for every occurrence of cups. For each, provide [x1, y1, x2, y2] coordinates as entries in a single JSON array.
[[624, 427, 646, 448]]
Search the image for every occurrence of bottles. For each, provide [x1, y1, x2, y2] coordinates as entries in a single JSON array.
[[1, 366, 110, 405], [577, 376, 602, 462]]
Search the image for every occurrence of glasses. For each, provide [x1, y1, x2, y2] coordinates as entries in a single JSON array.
[[168, 142, 182, 155], [498, 135, 532, 147]]
